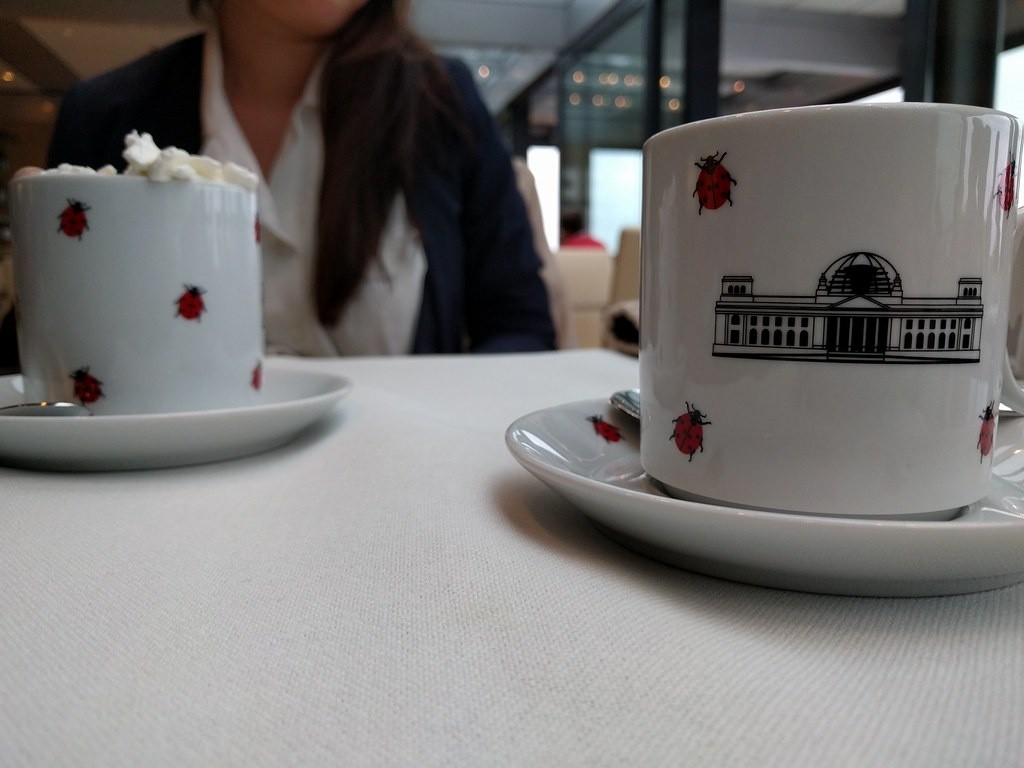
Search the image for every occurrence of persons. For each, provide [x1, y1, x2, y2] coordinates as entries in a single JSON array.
[[558, 212, 606, 252], [0, 0, 559, 378]]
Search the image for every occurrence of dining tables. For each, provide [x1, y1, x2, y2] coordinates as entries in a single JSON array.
[[0, 350, 1024, 768]]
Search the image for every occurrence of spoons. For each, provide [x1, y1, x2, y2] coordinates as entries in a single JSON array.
[[609, 387, 1024, 421], [0, 401, 96, 416]]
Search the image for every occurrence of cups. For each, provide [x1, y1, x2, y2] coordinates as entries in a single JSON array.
[[10, 174, 266, 415], [639, 101, 1024, 513]]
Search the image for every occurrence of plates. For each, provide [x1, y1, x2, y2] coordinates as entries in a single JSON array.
[[0, 363, 350, 471], [506, 394, 1024, 601]]
